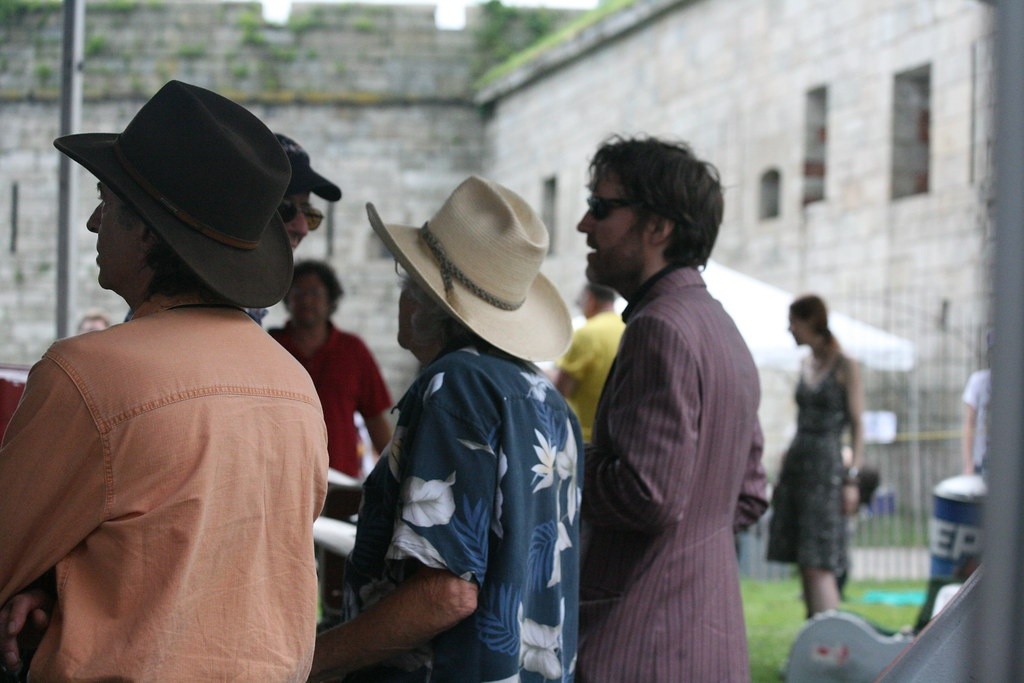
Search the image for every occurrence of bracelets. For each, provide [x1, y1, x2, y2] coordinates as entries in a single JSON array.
[[843, 477, 860, 485]]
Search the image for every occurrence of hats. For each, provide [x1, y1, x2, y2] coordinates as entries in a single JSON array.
[[53, 80, 292, 308], [273, 131, 341, 201], [366, 175, 572, 362], [586, 282, 615, 299]]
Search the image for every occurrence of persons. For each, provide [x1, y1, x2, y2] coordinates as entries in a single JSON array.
[[962, 333, 992, 476], [544, 283, 626, 447], [310, 178, 585, 683], [768, 295, 880, 618], [0, 80, 392, 683], [577, 134, 768, 683]]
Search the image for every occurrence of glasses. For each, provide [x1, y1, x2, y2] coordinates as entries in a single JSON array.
[[585, 197, 663, 220], [275, 200, 326, 232]]
